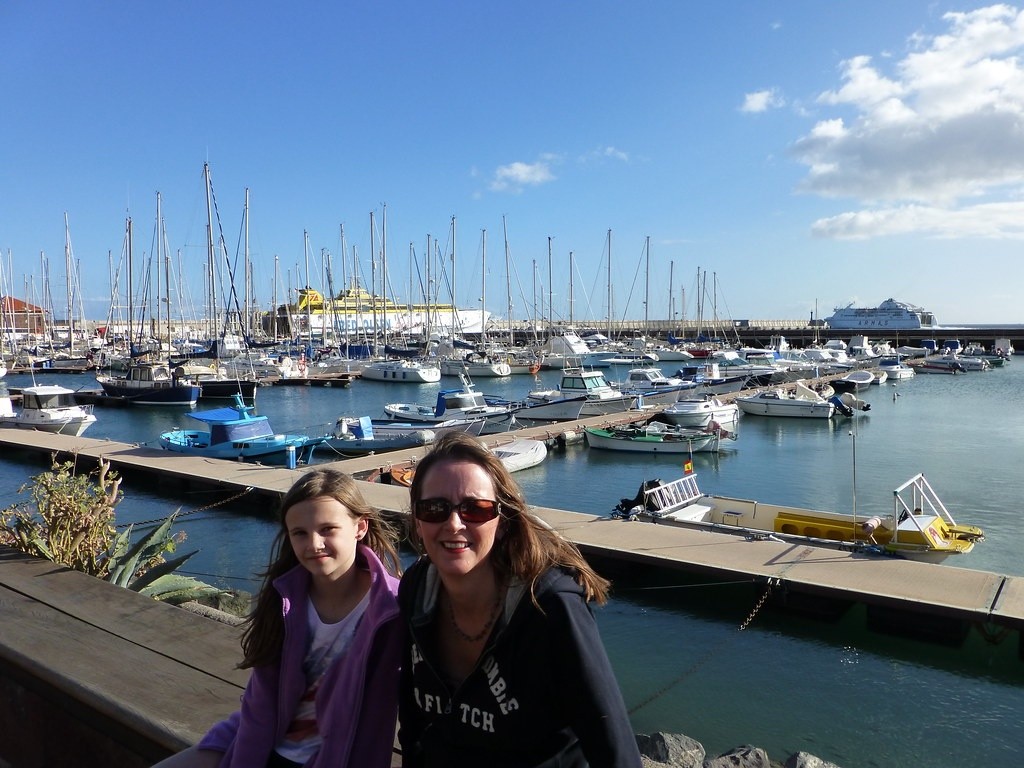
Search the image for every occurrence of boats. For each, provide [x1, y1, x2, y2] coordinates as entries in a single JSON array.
[[311, 416, 437, 454], [482, 390, 590, 422], [739, 389, 871, 419], [382, 375, 519, 436], [254, 275, 492, 335], [524, 354, 637, 413], [582, 425, 722, 454], [158, 389, 335, 464], [660, 398, 739, 428], [332, 415, 488, 441], [390, 437, 549, 488], [612, 437, 985, 564], [0, 384, 98, 437]]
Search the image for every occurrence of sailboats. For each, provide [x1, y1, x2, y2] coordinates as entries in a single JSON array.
[[96, 189, 202, 407], [0, 161, 1014, 401]]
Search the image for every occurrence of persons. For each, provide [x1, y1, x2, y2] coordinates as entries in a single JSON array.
[[151, 467, 401, 767], [396, 429, 643, 768]]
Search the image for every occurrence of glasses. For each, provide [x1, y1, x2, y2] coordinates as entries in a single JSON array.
[[413, 498, 502, 523]]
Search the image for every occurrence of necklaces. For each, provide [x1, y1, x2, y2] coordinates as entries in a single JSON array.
[[444, 586, 502, 643]]
